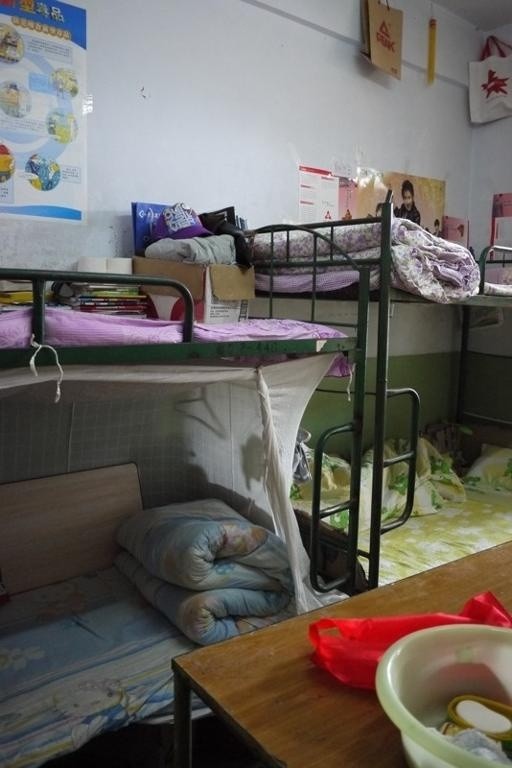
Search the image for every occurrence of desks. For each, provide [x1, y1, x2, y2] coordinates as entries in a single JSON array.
[[170, 541, 511, 768]]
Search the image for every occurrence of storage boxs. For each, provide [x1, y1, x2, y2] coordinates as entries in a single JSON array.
[[133, 255, 255, 322]]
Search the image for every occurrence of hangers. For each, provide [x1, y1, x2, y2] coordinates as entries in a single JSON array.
[[170, 388, 226, 438]]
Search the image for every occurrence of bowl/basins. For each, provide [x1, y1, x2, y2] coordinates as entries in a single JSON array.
[[376, 623, 512, 768]]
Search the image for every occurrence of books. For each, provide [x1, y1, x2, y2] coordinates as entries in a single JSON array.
[[66, 280, 149, 322]]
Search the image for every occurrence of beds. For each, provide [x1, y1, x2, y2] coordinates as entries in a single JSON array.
[[215, 190, 512, 592], [2, 224, 370, 768]]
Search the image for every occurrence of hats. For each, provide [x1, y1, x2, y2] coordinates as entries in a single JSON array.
[[155, 201, 213, 239]]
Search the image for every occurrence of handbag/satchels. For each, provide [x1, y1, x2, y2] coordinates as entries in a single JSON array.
[[293, 429, 313, 481]]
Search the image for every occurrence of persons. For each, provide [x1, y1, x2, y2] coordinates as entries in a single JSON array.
[[390, 180, 421, 227], [431, 218, 441, 236]]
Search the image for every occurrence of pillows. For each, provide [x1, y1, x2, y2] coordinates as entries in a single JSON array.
[[443, 438, 512, 496]]
